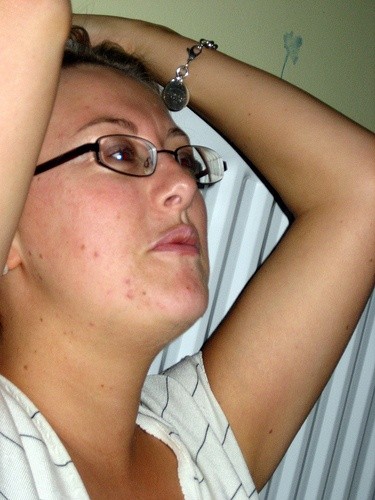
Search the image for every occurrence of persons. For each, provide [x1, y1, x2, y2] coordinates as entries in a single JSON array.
[[1, 0, 374, 500]]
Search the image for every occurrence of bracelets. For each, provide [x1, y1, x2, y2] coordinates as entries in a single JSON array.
[[162, 37, 218, 112]]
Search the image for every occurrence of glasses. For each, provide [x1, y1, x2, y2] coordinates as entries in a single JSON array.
[[34, 134, 228, 186]]
